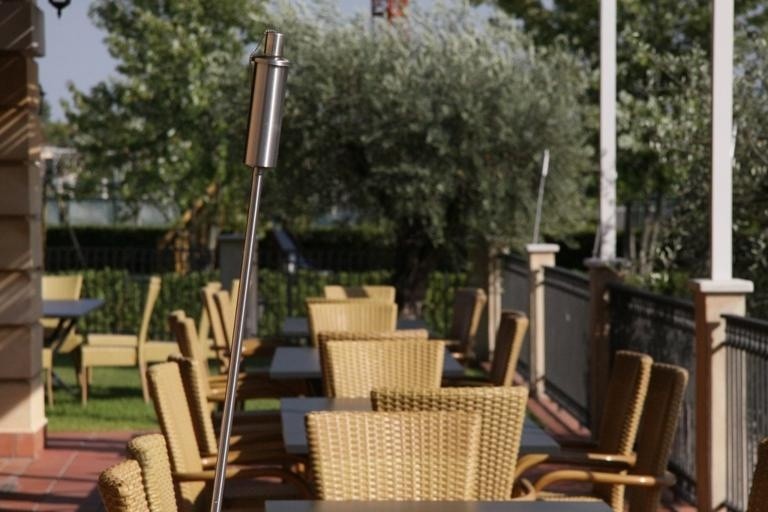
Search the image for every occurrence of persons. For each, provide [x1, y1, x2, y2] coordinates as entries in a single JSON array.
[[257, 213, 298, 274]]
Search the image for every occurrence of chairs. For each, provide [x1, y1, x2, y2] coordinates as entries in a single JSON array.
[[96, 274, 767, 511], [36, 274, 105, 410], [77, 274, 162, 407]]
